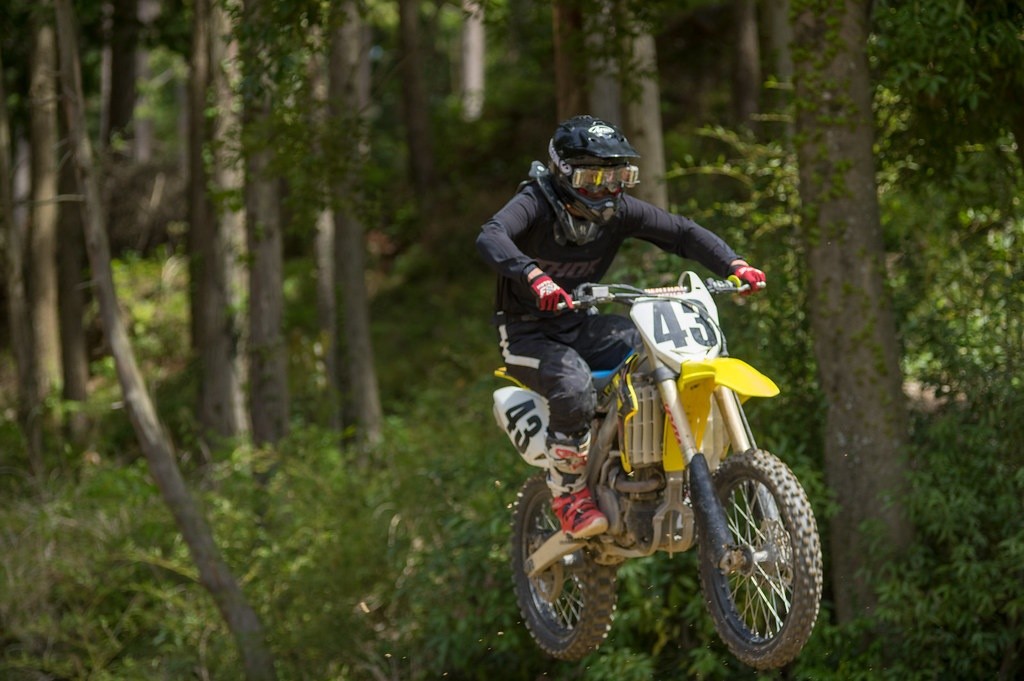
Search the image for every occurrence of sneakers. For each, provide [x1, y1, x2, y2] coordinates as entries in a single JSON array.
[[551, 488, 609, 539]]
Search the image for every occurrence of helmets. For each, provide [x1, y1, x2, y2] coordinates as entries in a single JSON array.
[[547, 115, 641, 224]]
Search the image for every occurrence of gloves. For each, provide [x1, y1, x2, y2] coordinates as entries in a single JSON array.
[[530, 272, 574, 313], [730, 264, 766, 295]]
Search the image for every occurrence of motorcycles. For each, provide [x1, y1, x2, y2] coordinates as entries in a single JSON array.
[[491, 274, 826, 670]]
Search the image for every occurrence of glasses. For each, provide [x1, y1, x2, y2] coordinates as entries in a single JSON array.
[[571, 166, 640, 194]]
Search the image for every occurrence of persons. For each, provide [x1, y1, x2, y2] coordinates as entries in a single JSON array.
[[475, 115, 767, 539]]
